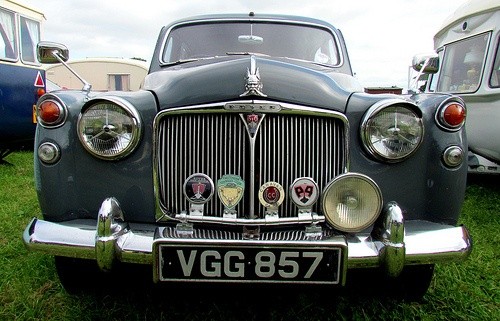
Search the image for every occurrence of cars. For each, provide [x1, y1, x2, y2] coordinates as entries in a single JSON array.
[[422, 0, 500, 174], [21, 11, 474, 298], [0, 0, 46, 156]]
